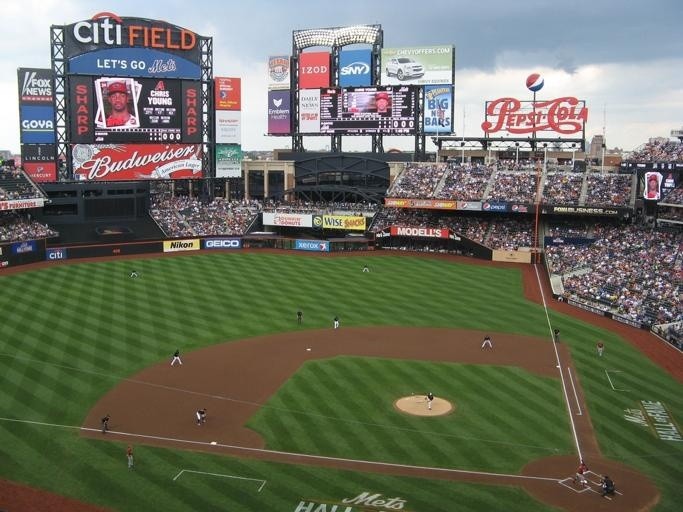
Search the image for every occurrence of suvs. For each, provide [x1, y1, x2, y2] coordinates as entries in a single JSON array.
[[385, 56, 424, 80]]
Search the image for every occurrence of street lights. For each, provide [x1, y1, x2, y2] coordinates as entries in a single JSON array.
[[533, 158, 541, 263]]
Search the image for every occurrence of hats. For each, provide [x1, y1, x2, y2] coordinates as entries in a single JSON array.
[[109, 83, 126, 93], [376, 93, 390, 100]]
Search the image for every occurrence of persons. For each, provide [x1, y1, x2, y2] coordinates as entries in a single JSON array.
[[129, 268, 136, 277], [362, 262, 368, 273], [374, 92, 393, 117], [425, 391, 434, 411], [296, 306, 304, 324], [573, 460, 588, 487], [0, 161, 59, 248], [196, 406, 207, 425], [481, 334, 493, 348], [101, 412, 111, 432], [126, 443, 135, 469], [169, 348, 185, 368], [547, 257, 682, 353], [104, 82, 138, 131], [333, 314, 339, 329], [599, 475, 616, 499]]
